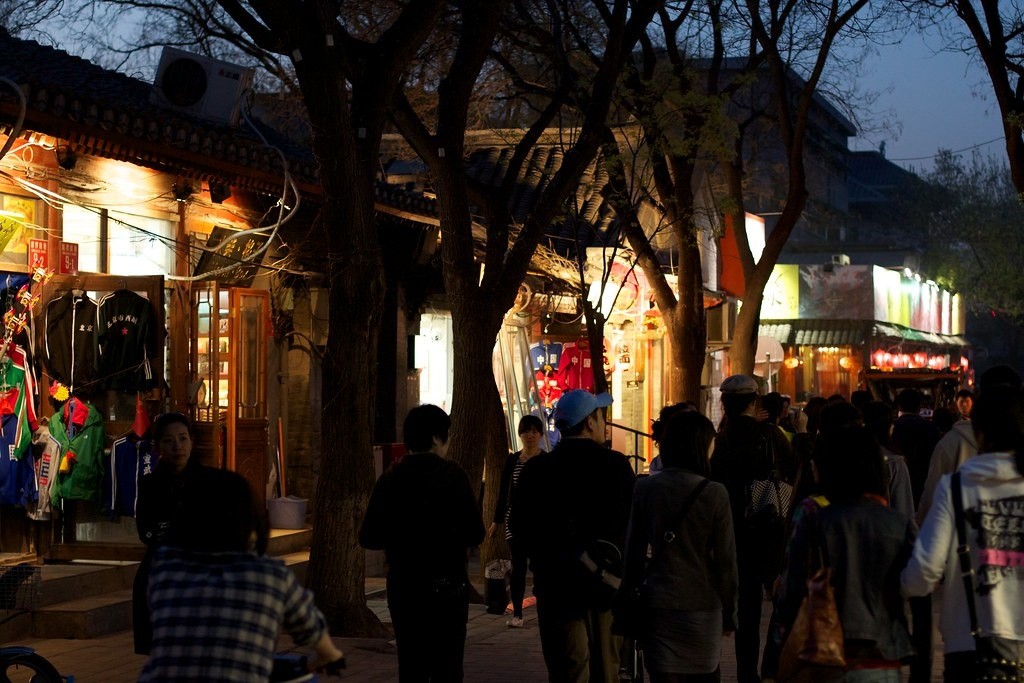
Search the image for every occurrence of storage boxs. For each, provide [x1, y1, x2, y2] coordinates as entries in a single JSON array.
[[485, 559, 512, 615]]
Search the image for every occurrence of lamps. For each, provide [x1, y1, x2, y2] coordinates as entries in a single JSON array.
[[208, 181, 231, 204], [839, 347, 852, 368], [547, 282, 558, 295], [784, 346, 798, 368], [173, 179, 193, 203], [53, 144, 76, 171], [650, 299, 655, 309]]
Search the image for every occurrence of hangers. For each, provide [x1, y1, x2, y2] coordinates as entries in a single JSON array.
[[71, 278, 87, 304], [540, 329, 595, 435]]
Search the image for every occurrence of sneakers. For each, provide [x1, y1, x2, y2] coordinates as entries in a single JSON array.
[[506, 616, 524, 627]]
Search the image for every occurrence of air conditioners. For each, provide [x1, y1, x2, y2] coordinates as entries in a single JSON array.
[[832, 254, 850, 265], [147, 46, 257, 129]]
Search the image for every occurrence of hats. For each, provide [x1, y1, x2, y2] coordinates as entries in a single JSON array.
[[554, 389, 612, 431], [719, 375, 761, 399]]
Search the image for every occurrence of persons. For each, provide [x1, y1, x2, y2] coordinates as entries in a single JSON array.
[[508, 390, 637, 682], [357, 405, 485, 683], [136, 466, 343, 683], [488, 415, 547, 627], [132, 414, 201, 654], [617, 376, 1024, 683]]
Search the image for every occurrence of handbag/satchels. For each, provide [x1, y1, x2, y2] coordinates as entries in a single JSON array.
[[748, 423, 794, 526], [483, 535, 512, 615], [610, 584, 654, 641], [774, 505, 847, 683], [973, 629, 1024, 682], [583, 538, 624, 593]]
